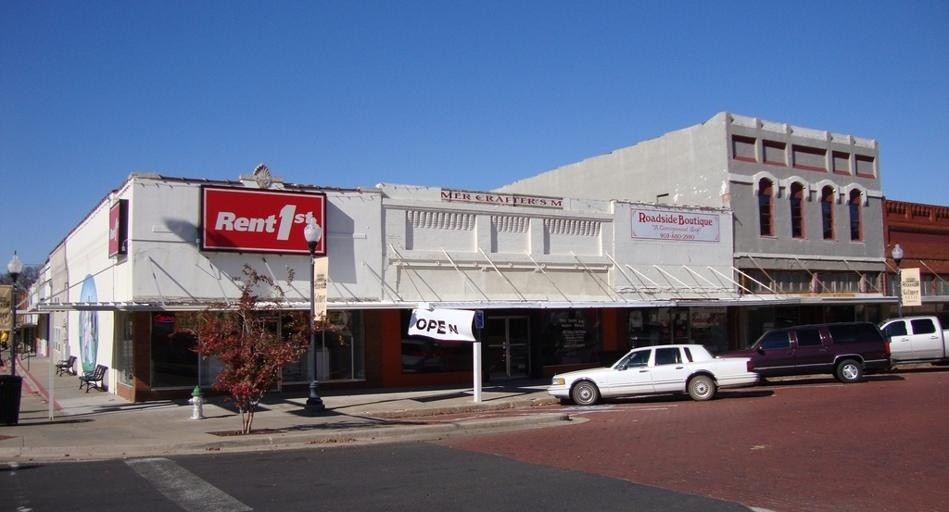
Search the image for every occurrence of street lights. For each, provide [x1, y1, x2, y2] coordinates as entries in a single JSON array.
[[304, 217, 325, 410], [892, 244, 904, 318], [8, 251, 23, 376]]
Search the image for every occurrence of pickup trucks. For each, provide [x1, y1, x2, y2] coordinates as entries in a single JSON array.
[[879, 315, 949, 364]]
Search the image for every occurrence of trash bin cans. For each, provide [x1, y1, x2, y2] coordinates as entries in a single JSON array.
[[0, 375, 22, 426]]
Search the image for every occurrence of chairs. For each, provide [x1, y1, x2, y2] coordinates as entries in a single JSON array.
[[78, 364, 108, 392], [55, 355, 77, 377]]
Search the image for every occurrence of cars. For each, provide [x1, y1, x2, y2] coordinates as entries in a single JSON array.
[[546, 344, 760, 405]]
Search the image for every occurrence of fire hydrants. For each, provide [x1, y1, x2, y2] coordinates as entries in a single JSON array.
[[188, 385, 208, 419]]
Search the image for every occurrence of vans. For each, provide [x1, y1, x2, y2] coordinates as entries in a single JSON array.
[[715, 322, 891, 383]]
